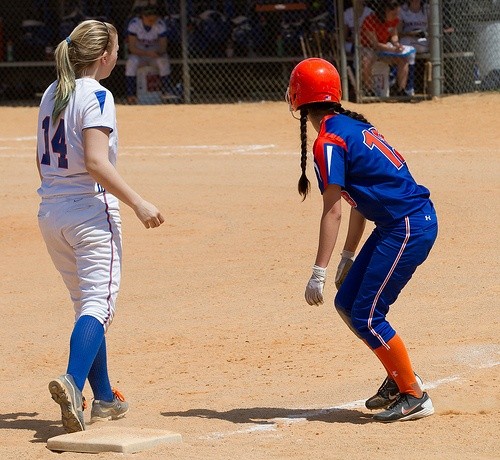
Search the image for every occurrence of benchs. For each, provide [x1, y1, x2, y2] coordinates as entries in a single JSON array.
[[0, 52, 474, 102]]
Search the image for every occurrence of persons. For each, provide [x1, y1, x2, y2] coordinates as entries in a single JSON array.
[[24, 0, 112, 50], [123, 6, 180, 103], [189, 0, 455, 101], [286, 58, 437, 424], [37, 19, 165, 435]]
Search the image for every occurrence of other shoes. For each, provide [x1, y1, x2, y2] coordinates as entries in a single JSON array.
[[365, 89, 375, 97], [128, 95, 137, 102], [397, 89, 408, 96], [162, 91, 181, 98]]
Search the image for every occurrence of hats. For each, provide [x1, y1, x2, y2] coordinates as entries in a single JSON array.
[[143, 7, 156, 16]]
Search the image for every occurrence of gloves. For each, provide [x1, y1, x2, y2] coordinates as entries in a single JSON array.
[[305, 264, 327, 307], [335, 250, 355, 290]]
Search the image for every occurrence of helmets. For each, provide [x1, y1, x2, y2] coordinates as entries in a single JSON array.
[[285, 58, 341, 120]]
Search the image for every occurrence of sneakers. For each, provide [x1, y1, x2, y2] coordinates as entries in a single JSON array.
[[48, 374, 87, 433], [372, 391, 435, 422], [91, 389, 129, 422], [366, 371, 423, 410]]
